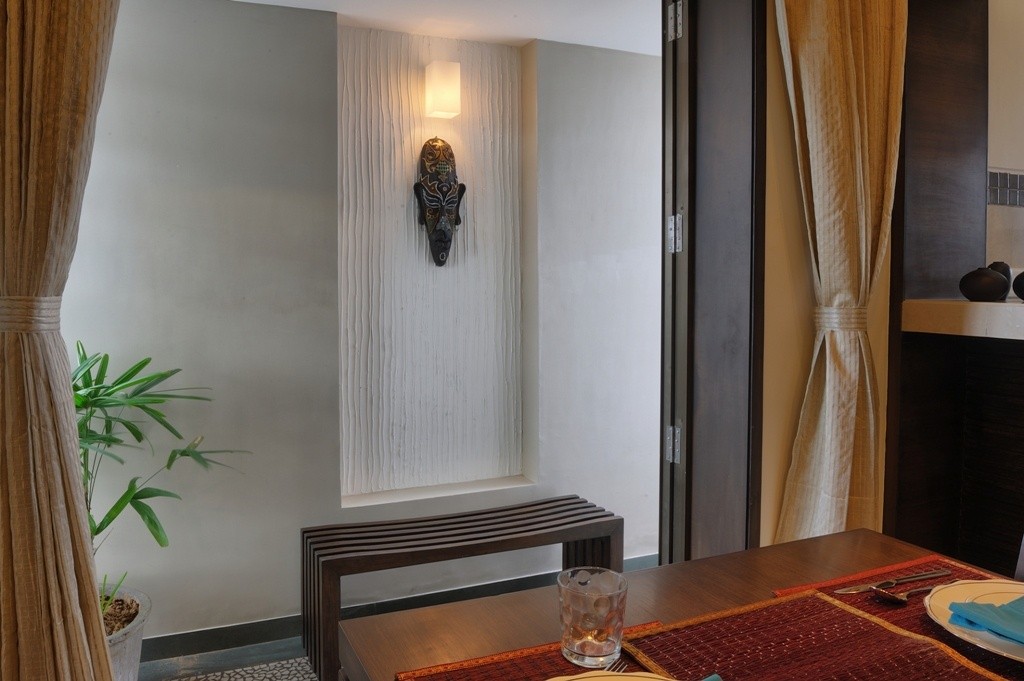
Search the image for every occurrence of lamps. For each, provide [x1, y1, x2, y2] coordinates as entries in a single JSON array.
[[413, 59, 465, 267]]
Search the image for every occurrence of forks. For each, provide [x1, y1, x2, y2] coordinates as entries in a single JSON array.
[[868, 585, 934, 602], [603, 657, 628, 672]]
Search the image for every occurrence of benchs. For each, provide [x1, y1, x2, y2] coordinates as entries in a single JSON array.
[[300, 494, 624, 681]]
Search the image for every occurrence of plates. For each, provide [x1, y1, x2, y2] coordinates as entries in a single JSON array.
[[923, 579, 1024, 664], [546, 670, 681, 681]]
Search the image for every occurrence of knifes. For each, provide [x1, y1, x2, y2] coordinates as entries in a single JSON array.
[[833, 569, 952, 594]]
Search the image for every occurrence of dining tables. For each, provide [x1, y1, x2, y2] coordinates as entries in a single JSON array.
[[337, 527, 1024, 681]]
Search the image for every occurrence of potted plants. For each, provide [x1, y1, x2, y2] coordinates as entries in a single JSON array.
[[69, 340, 254, 681]]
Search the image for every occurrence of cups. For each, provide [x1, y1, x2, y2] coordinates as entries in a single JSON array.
[[556, 566, 627, 668]]
